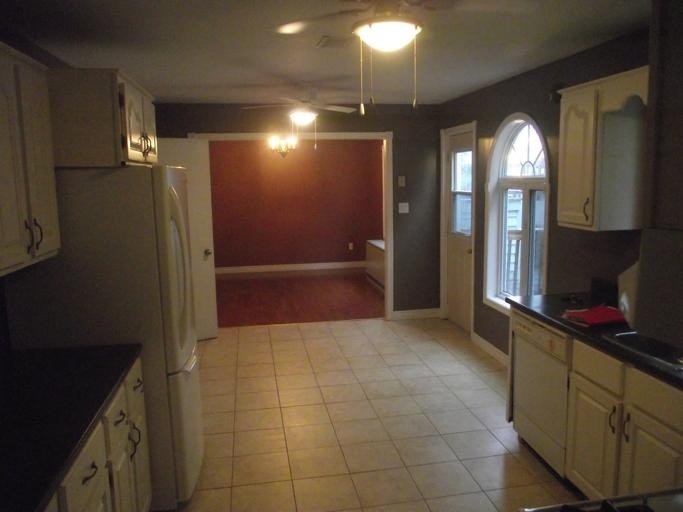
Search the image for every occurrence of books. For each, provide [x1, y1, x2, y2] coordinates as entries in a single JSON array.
[[563, 305, 626, 329]]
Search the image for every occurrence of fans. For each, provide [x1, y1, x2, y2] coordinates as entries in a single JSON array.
[[269, 1, 538, 39]]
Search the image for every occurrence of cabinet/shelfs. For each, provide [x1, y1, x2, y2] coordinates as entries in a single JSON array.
[[56, 421, 111, 510], [49, 66, 158, 166], [568, 341, 683, 512], [554, 66, 650, 233], [100, 356, 155, 510], [0, 37, 65, 280]]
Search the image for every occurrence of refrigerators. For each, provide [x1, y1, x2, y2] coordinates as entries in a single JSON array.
[[29, 165, 205, 511]]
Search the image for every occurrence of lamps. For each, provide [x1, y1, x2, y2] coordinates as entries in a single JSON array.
[[347, 7, 427, 118], [287, 107, 319, 150], [268, 136, 298, 159], [238, 94, 358, 116]]
